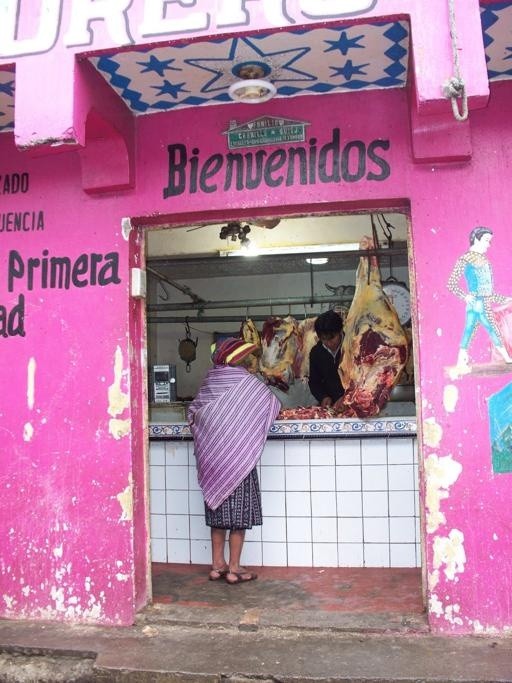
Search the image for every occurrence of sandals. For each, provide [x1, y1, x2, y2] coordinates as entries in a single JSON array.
[[226, 569, 258, 584], [209, 565, 229, 581]]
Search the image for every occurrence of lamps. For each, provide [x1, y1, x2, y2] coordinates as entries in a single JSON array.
[[220, 222, 254, 256], [227, 61, 277, 104]]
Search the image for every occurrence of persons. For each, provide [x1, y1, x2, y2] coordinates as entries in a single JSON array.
[[308, 310, 347, 407], [186, 337, 282, 582], [446, 227, 512, 368]]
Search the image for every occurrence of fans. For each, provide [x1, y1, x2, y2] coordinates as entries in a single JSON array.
[[186, 219, 282, 230]]
